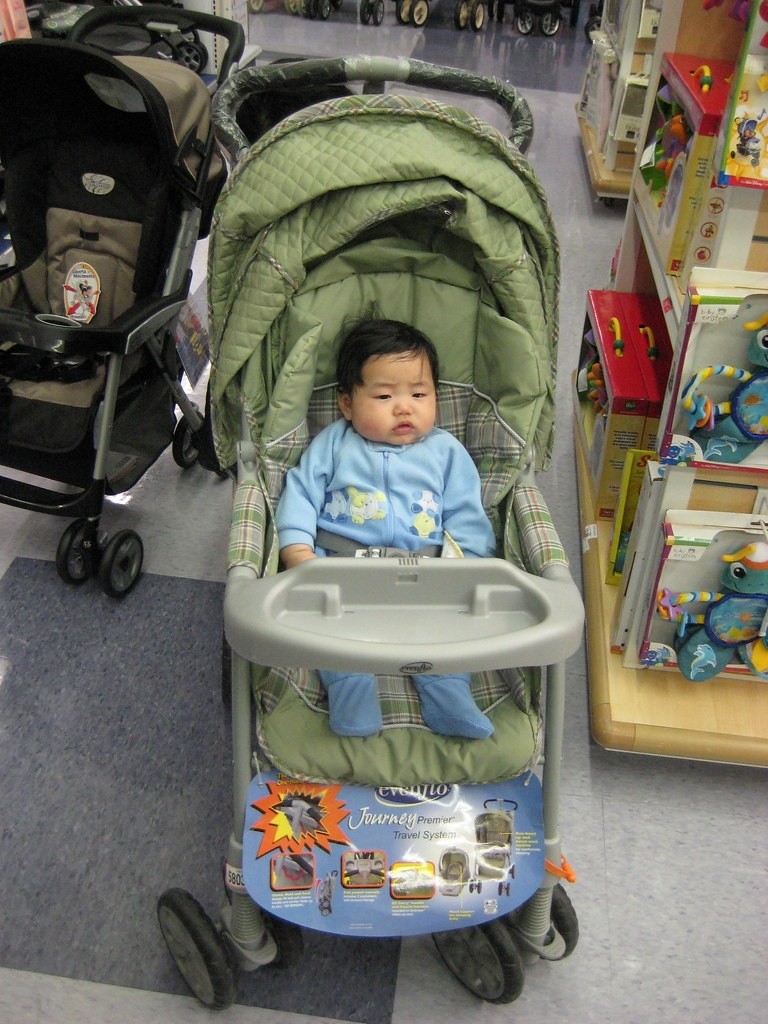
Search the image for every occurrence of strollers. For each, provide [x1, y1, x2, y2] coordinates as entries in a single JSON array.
[[157, 53, 586, 1013], [0, 1, 249, 606], [242, 0, 604, 46]]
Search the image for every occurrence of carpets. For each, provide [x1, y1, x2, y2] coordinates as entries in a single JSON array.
[[0, 556, 401, 1023]]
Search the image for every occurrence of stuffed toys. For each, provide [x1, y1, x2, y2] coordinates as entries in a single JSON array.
[[638, 83, 694, 197], [656, 540, 768, 683], [576, 328, 609, 431], [681, 310, 768, 464]]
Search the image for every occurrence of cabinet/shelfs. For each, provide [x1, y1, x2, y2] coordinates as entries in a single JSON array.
[[577, 0, 768, 768], [577, 0, 663, 204]]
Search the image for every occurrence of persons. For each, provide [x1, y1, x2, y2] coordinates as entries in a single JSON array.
[[274, 301, 497, 742]]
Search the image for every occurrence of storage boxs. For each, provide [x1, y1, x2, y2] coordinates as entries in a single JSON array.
[[576, 53, 768, 683]]
